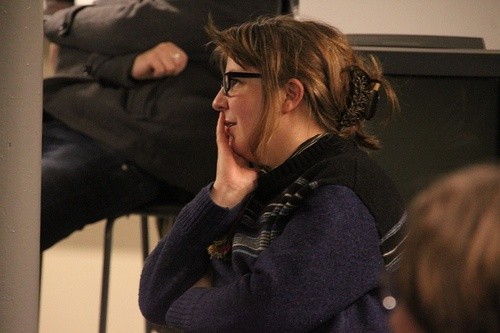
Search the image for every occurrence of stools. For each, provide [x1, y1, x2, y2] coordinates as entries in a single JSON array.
[[98, 206, 186, 333]]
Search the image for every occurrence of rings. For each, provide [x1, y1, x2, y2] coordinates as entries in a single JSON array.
[[172, 53, 181, 60]]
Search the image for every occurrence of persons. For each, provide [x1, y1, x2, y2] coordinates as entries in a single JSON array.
[[40, 0, 299, 252], [139, 11, 407, 333], [381, 165, 500, 333]]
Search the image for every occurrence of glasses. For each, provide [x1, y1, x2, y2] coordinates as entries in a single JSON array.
[[222, 72, 307, 101]]
